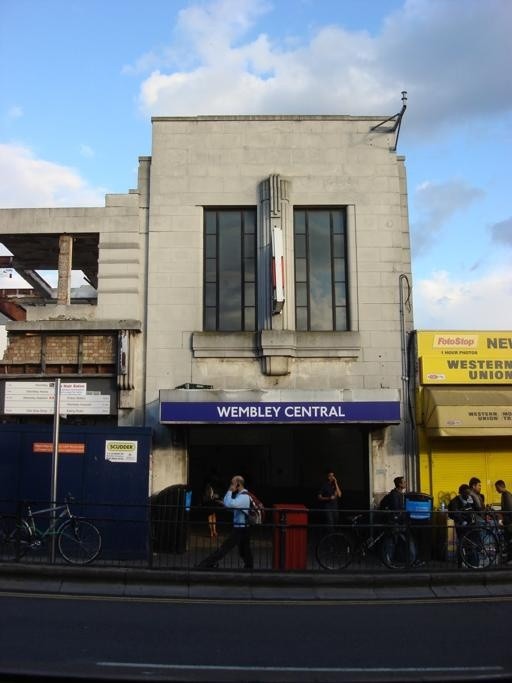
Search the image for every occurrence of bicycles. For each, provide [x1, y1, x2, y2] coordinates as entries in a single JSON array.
[[0, 493, 101, 564], [457, 511, 512, 572], [316, 513, 414, 570]]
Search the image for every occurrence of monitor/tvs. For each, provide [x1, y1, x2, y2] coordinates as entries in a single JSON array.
[[404, 494, 433, 523]]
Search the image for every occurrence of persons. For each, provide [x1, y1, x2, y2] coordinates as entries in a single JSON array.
[[495, 480, 512, 565], [200, 482, 218, 537], [469, 478, 487, 556], [198, 476, 255, 568], [382, 476, 426, 569], [448, 485, 484, 569], [313, 471, 342, 552]]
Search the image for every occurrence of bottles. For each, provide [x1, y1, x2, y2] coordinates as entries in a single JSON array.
[[441, 502, 445, 511]]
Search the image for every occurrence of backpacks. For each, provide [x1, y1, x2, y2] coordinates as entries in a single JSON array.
[[235, 490, 266, 525]]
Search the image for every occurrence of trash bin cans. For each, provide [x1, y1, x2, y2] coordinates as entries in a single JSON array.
[[272, 504, 310, 571], [153, 484, 194, 555], [405, 493, 434, 563]]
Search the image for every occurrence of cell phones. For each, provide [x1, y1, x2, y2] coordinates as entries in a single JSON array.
[[236, 484, 240, 488]]
